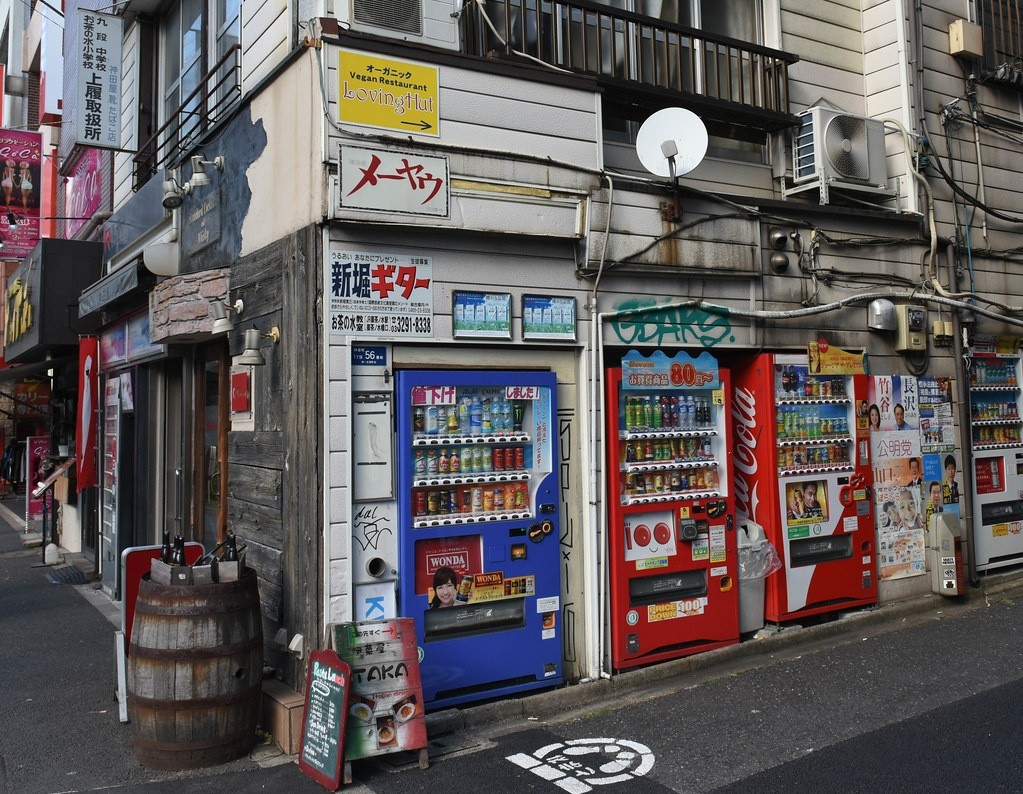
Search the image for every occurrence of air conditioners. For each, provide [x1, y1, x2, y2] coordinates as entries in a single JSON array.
[[334, 0, 461, 51], [791, 106, 890, 188]]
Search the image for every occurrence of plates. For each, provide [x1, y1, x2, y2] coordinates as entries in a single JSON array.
[[350, 703, 372, 721], [377, 726, 395, 742], [396, 703, 415, 722]]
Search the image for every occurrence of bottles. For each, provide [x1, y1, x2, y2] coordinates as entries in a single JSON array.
[[226, 530, 238, 561], [970, 363, 1017, 384], [972, 401, 1017, 420], [159, 530, 185, 565], [781, 365, 799, 392], [413, 396, 513, 433], [625, 396, 712, 428], [777, 406, 820, 437], [438, 449, 460, 473]]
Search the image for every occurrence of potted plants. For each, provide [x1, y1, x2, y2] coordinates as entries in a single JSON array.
[[51, 414, 75, 457]]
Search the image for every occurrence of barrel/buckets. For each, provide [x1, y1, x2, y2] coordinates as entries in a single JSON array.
[[127, 572, 264, 770]]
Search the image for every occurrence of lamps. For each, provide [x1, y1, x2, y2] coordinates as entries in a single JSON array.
[[0, 236, 40, 249], [161, 177, 193, 210], [6, 210, 102, 230], [210, 290, 243, 335], [189, 155, 223, 187], [237, 322, 280, 365]]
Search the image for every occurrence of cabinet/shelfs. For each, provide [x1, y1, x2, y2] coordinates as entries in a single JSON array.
[[100, 400, 135, 599]]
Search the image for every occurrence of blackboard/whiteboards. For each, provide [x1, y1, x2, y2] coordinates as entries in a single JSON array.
[[288, 649, 351, 790]]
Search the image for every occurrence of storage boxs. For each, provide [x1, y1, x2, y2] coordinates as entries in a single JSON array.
[[193, 555, 217, 585], [150, 556, 192, 586], [217, 551, 246, 582]]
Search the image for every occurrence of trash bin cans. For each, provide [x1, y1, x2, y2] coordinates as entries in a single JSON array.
[[735, 513, 769, 633]]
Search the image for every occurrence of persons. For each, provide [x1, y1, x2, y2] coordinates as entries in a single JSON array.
[[790, 481, 823, 520], [895, 489, 925, 531], [429, 566, 468, 609], [19, 161, 33, 213], [941, 454, 959, 504], [926, 480, 943, 532], [868, 404, 885, 431], [0, 159, 18, 210], [882, 501, 901, 530], [893, 403, 912, 431], [858, 400, 869, 417], [905, 457, 922, 487]]
